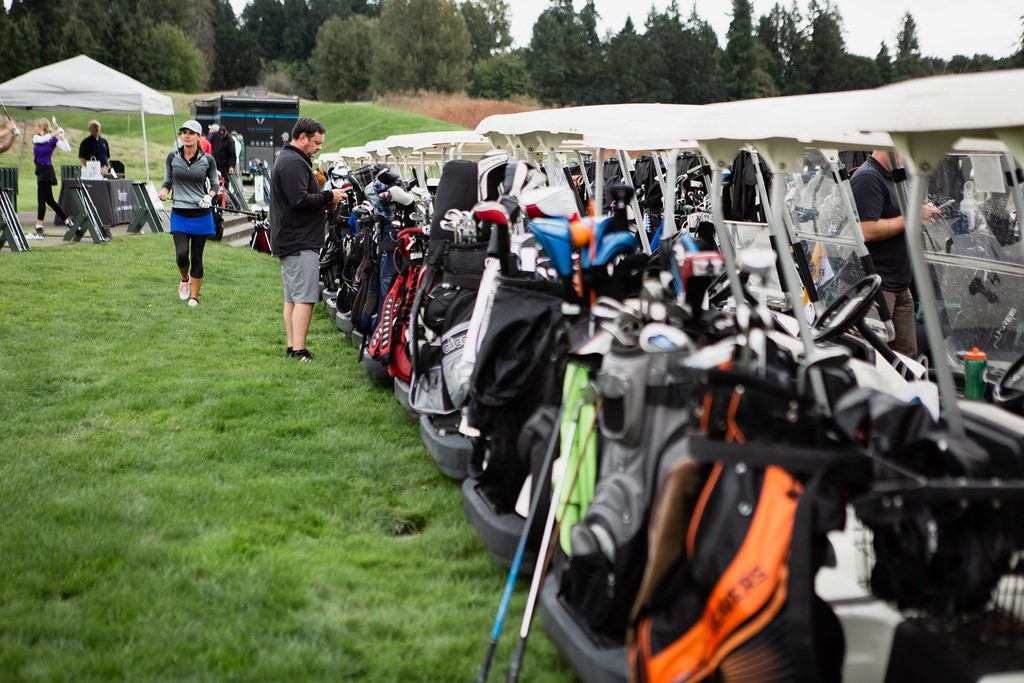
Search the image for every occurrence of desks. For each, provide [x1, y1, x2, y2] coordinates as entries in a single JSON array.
[[53, 180, 135, 227]]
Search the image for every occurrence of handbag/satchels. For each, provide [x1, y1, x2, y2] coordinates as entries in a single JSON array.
[[86, 156, 100, 179]]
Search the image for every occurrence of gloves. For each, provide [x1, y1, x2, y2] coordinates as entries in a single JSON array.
[[198, 195, 212, 208]]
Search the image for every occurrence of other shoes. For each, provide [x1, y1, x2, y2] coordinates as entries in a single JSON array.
[[67, 218, 74, 228], [34, 226, 44, 232]]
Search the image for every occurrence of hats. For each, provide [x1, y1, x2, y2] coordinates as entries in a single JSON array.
[[281, 132, 289, 138], [179, 119, 202, 135], [208, 123, 220, 132]]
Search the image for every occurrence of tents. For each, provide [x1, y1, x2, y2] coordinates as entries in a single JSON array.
[[0, 54, 179, 188]]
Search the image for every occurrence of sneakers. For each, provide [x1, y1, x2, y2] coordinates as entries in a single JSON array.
[[187, 298, 198, 306], [178, 274, 191, 300], [285, 348, 315, 362]]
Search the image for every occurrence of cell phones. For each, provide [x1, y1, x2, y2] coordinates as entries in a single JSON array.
[[938, 199, 956, 210], [345, 189, 354, 195]]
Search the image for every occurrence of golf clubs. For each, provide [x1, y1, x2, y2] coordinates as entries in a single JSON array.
[[734, 249, 869, 433], [74, 167, 110, 243], [0, 100, 22, 135], [0, 190, 26, 251], [160, 195, 257, 217], [591, 272, 733, 380], [408, 186, 432, 236], [51, 116, 63, 135], [440, 208, 480, 245], [332, 162, 348, 179], [511, 232, 560, 288]]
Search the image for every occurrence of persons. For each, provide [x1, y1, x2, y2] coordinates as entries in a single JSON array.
[[158, 120, 219, 305], [850, 150, 942, 357], [200, 124, 244, 193], [25, 117, 81, 240], [174, 132, 183, 149], [79, 120, 111, 173], [270, 118, 348, 361], [280, 131, 290, 146], [0, 121, 17, 154]]
